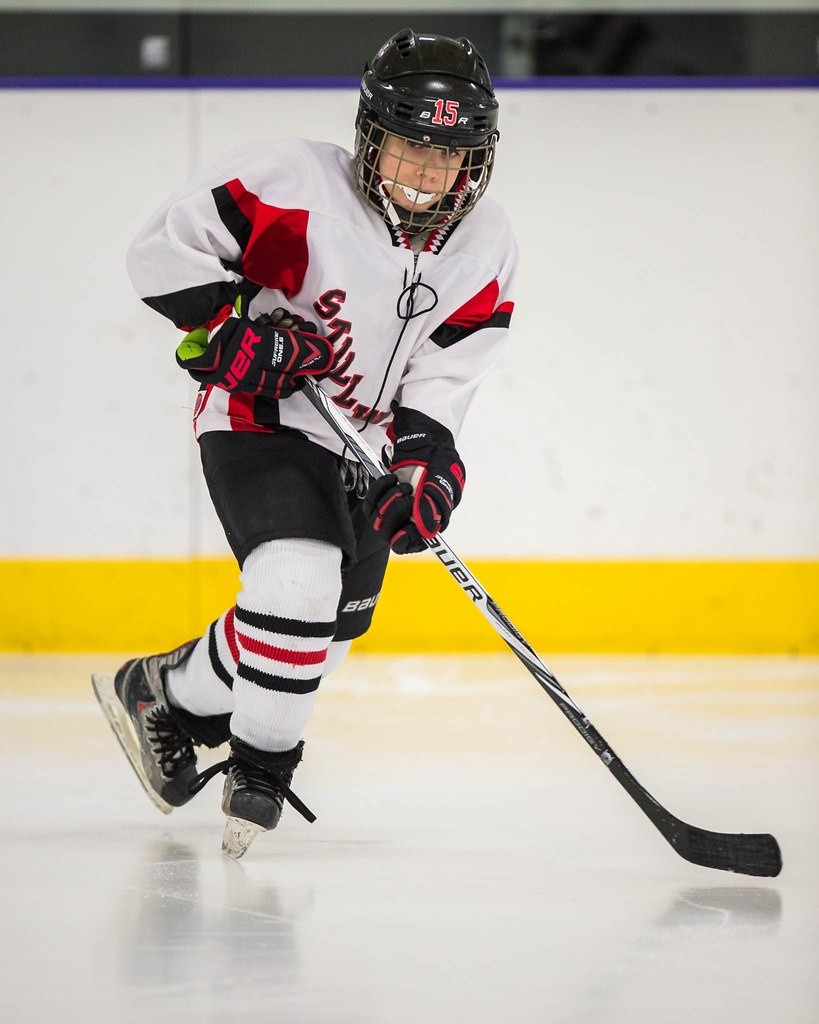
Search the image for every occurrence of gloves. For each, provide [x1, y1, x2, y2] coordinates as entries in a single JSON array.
[[362, 398, 467, 555], [175, 308, 335, 399]]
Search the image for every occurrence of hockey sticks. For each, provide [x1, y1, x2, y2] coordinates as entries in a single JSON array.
[[297, 370, 782, 878]]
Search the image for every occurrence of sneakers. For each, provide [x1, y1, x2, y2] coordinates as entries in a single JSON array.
[[207, 735, 317, 859], [91, 637, 232, 813]]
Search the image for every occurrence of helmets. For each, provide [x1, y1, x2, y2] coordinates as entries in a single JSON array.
[[353, 27, 499, 231]]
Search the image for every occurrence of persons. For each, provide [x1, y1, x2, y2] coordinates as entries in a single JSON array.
[[91, 29, 516, 860]]
